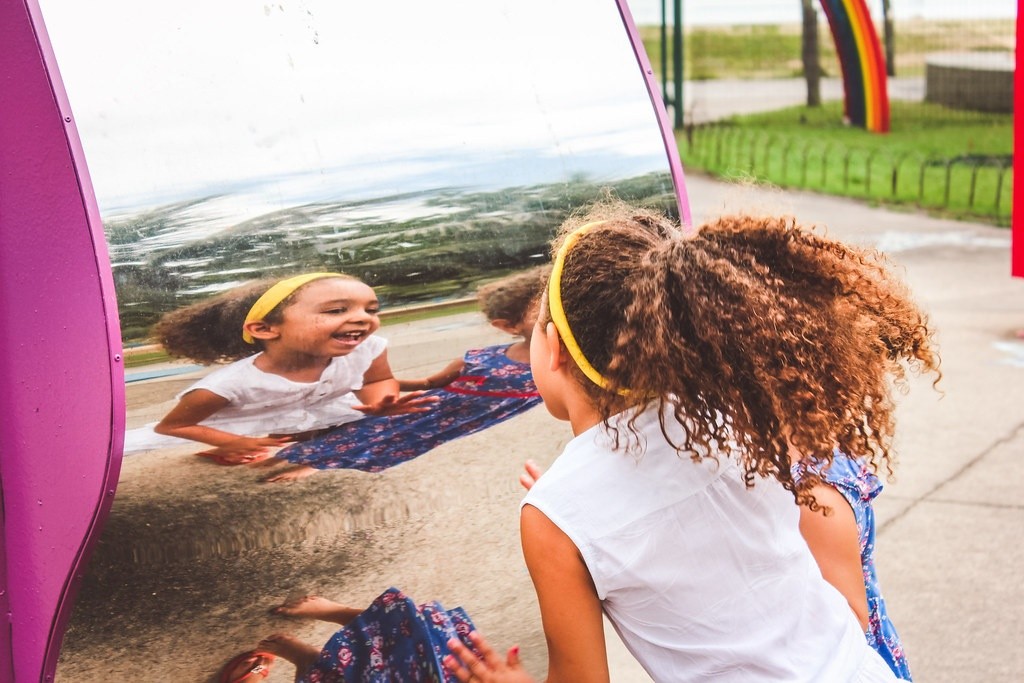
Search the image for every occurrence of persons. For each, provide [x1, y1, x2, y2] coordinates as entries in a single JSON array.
[[442, 186, 944, 683], [221, 587, 486, 683], [248, 261, 554, 483], [153, 272, 439, 467]]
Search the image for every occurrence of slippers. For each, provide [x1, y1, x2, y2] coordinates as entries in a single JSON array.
[[194, 450, 268, 465], [218, 651, 276, 683]]
